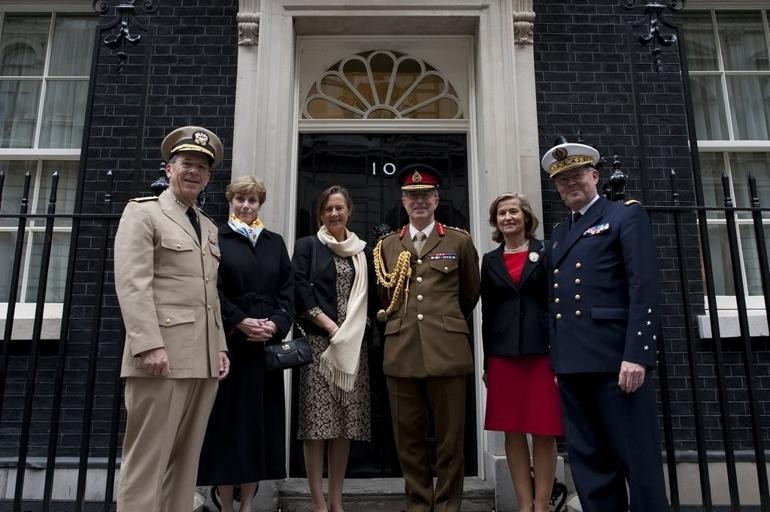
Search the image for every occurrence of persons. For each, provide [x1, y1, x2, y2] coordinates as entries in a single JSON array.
[[114, 124, 232, 512], [194, 177, 294, 511], [374, 164, 481, 510], [479, 191, 563, 510], [293, 184, 378, 512], [541, 141, 670, 511]]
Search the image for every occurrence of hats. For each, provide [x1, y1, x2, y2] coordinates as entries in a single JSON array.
[[541, 143, 601, 179], [159, 125, 225, 170], [397, 162, 444, 193]]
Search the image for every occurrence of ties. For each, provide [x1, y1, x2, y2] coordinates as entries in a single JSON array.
[[571, 212, 582, 231], [416, 232, 425, 256], [186, 206, 201, 243]]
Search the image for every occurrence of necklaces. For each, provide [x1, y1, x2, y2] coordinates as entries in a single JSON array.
[[504, 239, 530, 254]]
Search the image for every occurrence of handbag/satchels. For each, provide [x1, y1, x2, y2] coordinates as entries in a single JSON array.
[[262, 336, 313, 371]]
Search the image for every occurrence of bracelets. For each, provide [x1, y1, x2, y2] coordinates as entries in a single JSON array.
[[328, 327, 339, 337]]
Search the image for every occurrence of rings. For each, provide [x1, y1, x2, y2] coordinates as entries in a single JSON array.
[[633, 381, 639, 384]]
